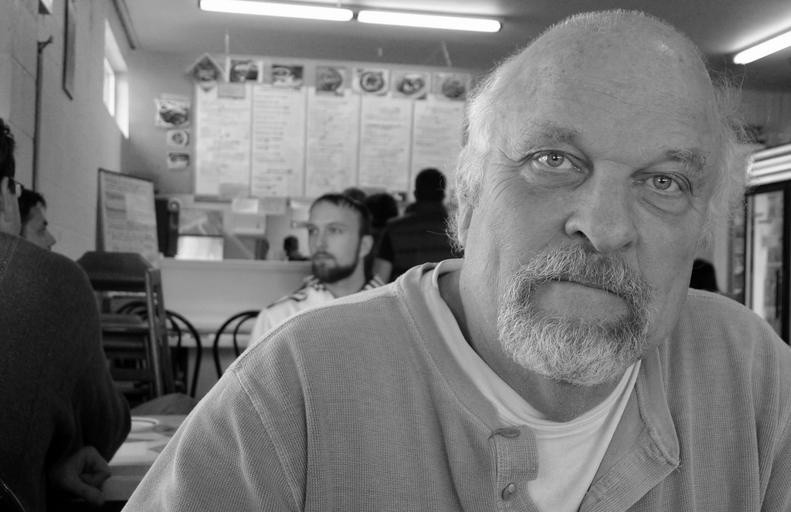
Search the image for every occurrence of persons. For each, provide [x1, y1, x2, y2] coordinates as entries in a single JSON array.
[[14, 181, 59, 250], [361, 192, 396, 278], [122, 6, 791, 512], [690, 257, 718, 292], [241, 192, 389, 350], [372, 164, 466, 286], [282, 236, 308, 261], [343, 187, 367, 201], [0, 119, 136, 512]]
